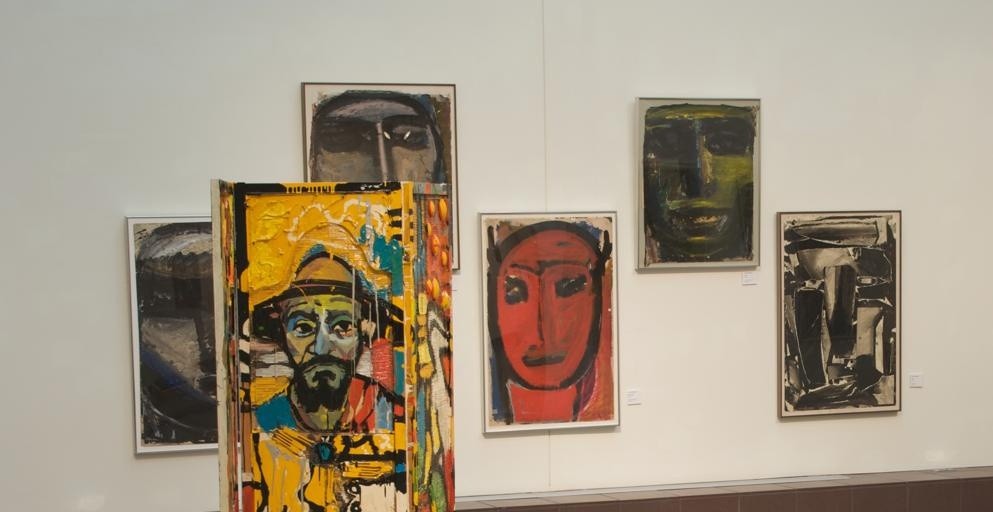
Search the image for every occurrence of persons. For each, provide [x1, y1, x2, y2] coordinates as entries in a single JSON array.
[[237, 244, 407, 511], [307, 87, 445, 184], [133, 219, 219, 447], [642, 101, 756, 264]]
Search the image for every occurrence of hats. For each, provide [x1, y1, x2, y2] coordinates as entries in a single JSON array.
[[253, 252, 403, 348]]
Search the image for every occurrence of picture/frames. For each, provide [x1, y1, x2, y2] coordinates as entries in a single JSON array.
[[776, 209, 902, 419], [634, 95, 763, 272], [123, 214, 220, 457], [300, 82, 461, 272]]
